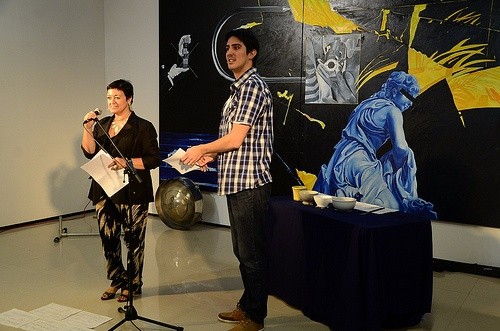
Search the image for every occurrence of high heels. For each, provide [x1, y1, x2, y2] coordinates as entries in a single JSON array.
[[101, 283, 133, 303]]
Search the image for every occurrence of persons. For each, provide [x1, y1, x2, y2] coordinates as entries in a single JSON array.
[[180, 28, 274, 331], [81, 79, 160, 302]]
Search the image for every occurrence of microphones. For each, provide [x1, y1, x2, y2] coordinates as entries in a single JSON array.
[[83, 108, 102, 124]]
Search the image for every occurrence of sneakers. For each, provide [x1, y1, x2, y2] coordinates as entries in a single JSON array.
[[217, 306, 247, 322], [227, 317, 264, 331]]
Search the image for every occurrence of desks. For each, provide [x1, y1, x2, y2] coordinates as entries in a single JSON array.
[[271, 196, 433, 331]]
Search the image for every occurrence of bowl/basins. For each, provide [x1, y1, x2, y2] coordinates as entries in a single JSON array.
[[300, 190, 319, 205], [332, 197, 356, 211], [312, 195, 332, 208]]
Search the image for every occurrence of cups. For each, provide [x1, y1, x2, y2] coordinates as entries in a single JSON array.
[[292, 186, 307, 201]]
